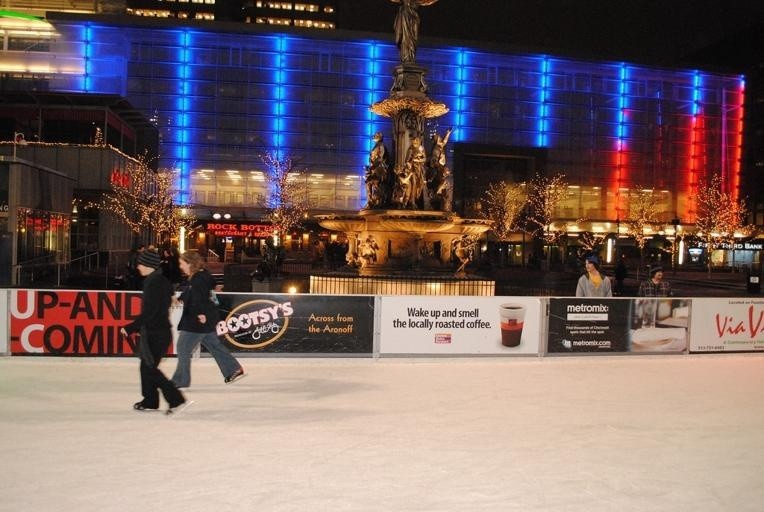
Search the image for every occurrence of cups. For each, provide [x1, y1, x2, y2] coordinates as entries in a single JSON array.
[[642, 301, 657, 332], [499, 304, 526, 348]]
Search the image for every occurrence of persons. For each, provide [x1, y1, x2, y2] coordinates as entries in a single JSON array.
[[364, 133, 389, 204], [453, 240, 478, 271], [575, 255, 612, 297], [393, 2, 419, 61], [638, 266, 673, 296], [316, 241, 344, 272], [430, 129, 453, 191], [118, 245, 187, 413], [614, 260, 627, 289], [407, 129, 426, 206], [359, 242, 375, 266], [169, 246, 245, 388]]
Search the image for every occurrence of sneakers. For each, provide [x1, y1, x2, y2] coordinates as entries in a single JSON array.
[[134, 401, 159, 410], [166, 400, 188, 417], [170, 380, 191, 388], [223, 366, 244, 383]]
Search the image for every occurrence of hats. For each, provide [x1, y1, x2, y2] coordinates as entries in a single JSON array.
[[137, 249, 161, 270]]
